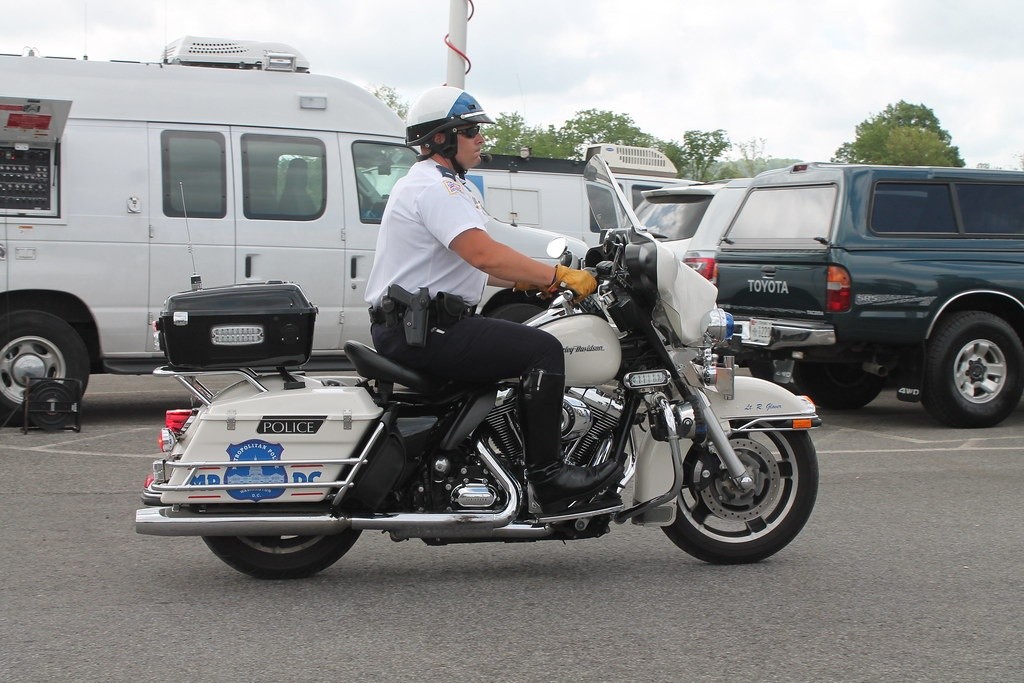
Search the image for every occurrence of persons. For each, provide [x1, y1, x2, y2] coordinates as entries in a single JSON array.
[[364, 85, 624, 513]]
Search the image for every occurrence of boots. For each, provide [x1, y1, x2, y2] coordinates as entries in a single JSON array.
[[517, 370, 625, 514]]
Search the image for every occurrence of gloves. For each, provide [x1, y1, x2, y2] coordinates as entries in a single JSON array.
[[513, 281, 558, 298], [552, 264, 597, 304]]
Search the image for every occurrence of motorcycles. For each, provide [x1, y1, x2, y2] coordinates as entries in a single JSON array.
[[133, 148, 822, 585]]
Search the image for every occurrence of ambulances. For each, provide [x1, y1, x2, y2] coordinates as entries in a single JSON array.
[[0, 38, 591, 428]]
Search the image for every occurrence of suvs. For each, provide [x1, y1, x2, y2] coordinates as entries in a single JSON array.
[[583, 142, 707, 212], [718, 164, 1024, 427], [635, 178, 756, 291]]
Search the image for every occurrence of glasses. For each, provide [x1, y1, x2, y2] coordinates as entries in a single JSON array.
[[455, 126, 480, 138]]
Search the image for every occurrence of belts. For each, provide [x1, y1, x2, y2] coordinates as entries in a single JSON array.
[[367, 304, 477, 323]]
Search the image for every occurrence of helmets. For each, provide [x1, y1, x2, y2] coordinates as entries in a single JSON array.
[[405, 85, 496, 146]]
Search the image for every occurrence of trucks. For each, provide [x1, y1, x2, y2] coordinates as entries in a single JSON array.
[[464, 151, 626, 248]]
